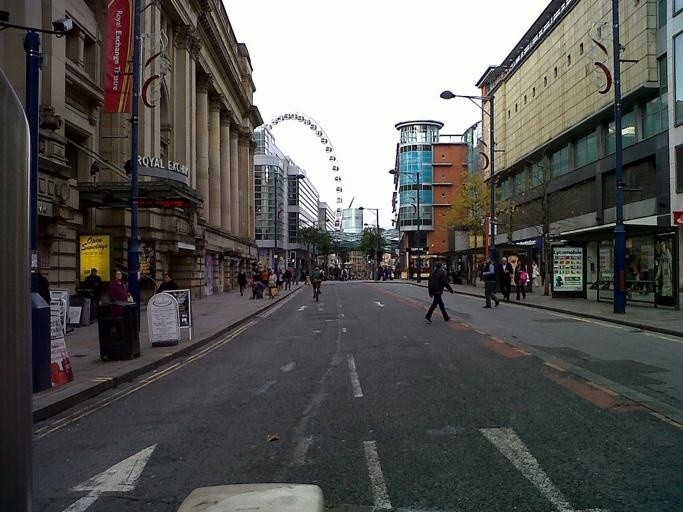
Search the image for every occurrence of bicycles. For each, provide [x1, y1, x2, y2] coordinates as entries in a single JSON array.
[[313, 281, 321, 301]]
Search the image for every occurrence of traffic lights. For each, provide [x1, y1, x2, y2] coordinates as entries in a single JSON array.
[[290, 251, 296, 262]]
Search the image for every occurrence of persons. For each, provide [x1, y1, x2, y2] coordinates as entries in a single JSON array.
[[82, 266, 101, 291], [531, 260, 540, 287], [109, 268, 131, 303], [480, 256, 500, 308], [624, 245, 655, 296], [377, 263, 401, 281], [236, 262, 351, 300], [656, 240, 674, 296], [655, 241, 670, 296], [424, 262, 453, 324], [498, 257, 513, 301], [156, 274, 181, 294], [513, 259, 527, 300]]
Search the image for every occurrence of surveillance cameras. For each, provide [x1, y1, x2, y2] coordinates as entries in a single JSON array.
[[52, 18, 73, 33]]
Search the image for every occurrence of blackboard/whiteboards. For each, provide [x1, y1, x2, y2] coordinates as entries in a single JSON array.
[[146, 289, 191, 343]]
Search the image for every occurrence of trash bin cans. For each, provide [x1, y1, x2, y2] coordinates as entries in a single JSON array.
[[96, 301, 140, 361], [31, 292, 52, 394], [76, 288, 94, 324], [453, 271, 462, 285]]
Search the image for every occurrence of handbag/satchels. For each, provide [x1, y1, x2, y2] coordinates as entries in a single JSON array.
[[271, 287, 280, 299], [268, 281, 276, 288]]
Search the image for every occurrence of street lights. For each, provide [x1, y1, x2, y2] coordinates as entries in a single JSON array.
[[313, 219, 330, 260], [389, 169, 420, 283], [359, 207, 379, 250], [441, 91, 495, 258], [275, 175, 305, 273]]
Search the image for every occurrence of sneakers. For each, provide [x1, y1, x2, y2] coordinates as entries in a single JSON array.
[[423, 317, 433, 324], [444, 315, 450, 322], [502, 295, 527, 301], [481, 300, 501, 311]]
